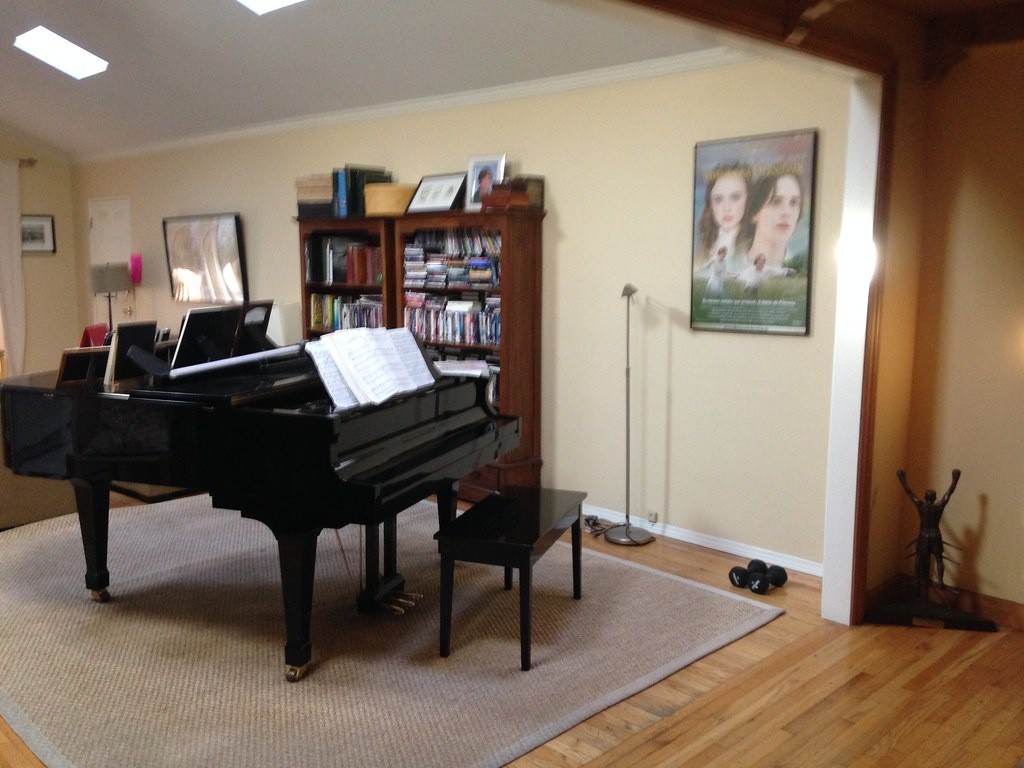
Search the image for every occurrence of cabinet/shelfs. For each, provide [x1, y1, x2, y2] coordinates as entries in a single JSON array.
[[394, 212, 546, 505], [288, 215, 393, 350]]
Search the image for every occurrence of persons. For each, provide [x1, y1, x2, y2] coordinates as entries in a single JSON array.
[[739, 254, 768, 292], [704, 247, 728, 293], [700, 160, 803, 276], [897, 469, 961, 589], [474, 166, 493, 203]]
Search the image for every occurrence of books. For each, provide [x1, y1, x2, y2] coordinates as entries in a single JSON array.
[[306, 236, 383, 330], [404, 226, 502, 345]]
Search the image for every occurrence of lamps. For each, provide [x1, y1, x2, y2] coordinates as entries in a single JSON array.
[[90, 262, 134, 331]]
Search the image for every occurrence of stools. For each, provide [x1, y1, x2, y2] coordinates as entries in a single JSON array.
[[434, 484, 588, 670]]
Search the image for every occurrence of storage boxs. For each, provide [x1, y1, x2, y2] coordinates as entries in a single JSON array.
[[363, 182, 413, 215], [297, 173, 333, 204]]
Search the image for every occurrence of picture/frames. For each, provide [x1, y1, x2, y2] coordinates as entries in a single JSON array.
[[465, 155, 505, 210], [404, 171, 466, 211], [21, 214, 55, 252], [163, 212, 249, 304]]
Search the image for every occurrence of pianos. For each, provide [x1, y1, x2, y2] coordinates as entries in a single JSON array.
[[0, 331, 523, 683]]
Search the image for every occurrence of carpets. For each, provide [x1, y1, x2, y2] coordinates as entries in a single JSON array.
[[0, 493, 789, 768]]
